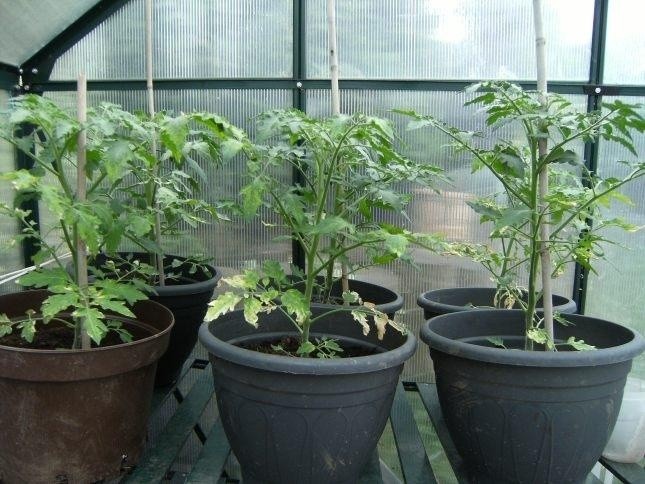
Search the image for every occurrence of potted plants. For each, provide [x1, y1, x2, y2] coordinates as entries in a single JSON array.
[[387, 79, 643, 484], [0, 79, 232, 482], [203, 106, 462, 482]]
